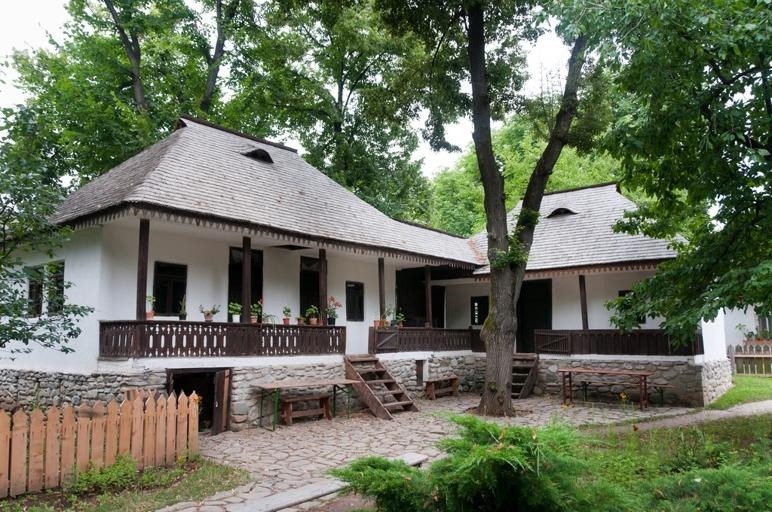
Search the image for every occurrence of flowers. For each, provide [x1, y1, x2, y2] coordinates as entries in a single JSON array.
[[324, 295, 343, 318]]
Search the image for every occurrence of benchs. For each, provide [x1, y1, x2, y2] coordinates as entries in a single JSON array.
[[427, 374, 459, 400], [578, 379, 674, 410], [280, 391, 335, 425]]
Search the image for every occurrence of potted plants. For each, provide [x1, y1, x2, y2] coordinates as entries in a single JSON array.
[[145, 296, 155, 320], [373, 306, 405, 328], [177, 294, 242, 323], [250, 298, 320, 326]]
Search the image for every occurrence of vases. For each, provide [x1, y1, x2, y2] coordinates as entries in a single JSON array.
[[327, 317, 337, 326]]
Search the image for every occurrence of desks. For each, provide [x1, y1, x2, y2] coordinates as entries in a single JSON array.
[[252, 379, 360, 433], [559, 366, 654, 409]]
